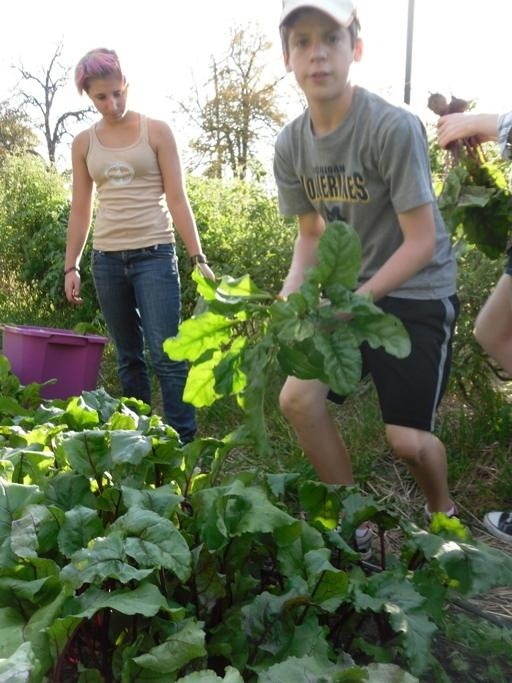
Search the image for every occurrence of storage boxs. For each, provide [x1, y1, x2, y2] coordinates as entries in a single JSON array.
[[0, 323, 109, 401]]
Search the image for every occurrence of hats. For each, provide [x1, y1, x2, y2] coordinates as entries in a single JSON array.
[[279, 0, 357, 30]]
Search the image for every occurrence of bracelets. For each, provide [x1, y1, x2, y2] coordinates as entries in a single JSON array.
[[63, 266, 82, 275], [190, 254, 208, 266]]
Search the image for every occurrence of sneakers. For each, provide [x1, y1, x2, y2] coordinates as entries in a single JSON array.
[[326, 527, 372, 562], [484, 511, 512, 541]]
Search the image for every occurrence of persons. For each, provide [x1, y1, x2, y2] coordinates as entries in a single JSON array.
[[54, 44, 226, 454], [256, 0, 463, 528], [433, 108, 512, 544]]
